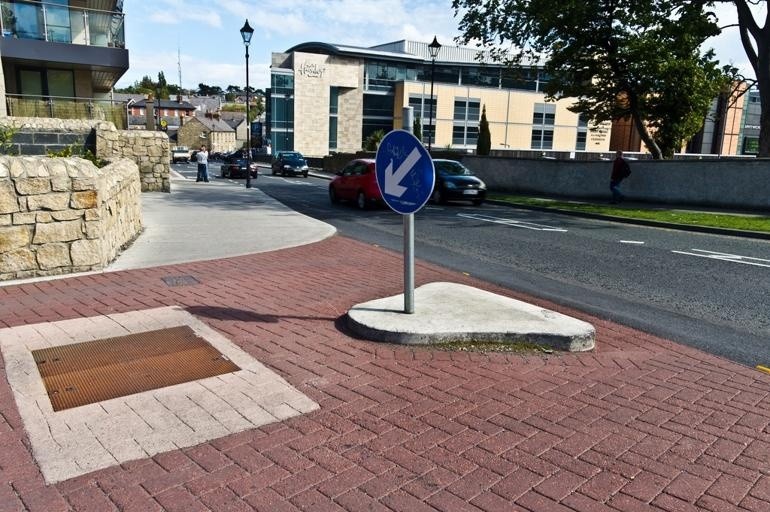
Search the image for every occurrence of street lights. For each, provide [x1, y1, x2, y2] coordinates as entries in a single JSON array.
[[241, 19, 254, 188], [428, 36, 442, 153]]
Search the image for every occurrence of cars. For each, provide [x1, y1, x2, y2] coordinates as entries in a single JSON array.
[[432, 159, 487, 204], [210, 148, 257, 160], [222, 160, 260, 177], [172, 146, 201, 163], [329, 158, 382, 209], [273, 150, 309, 177]]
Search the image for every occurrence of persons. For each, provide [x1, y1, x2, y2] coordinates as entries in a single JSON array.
[[6, 10, 17, 39], [265, 144, 273, 165], [609, 150, 631, 205], [197, 143, 211, 184]]
